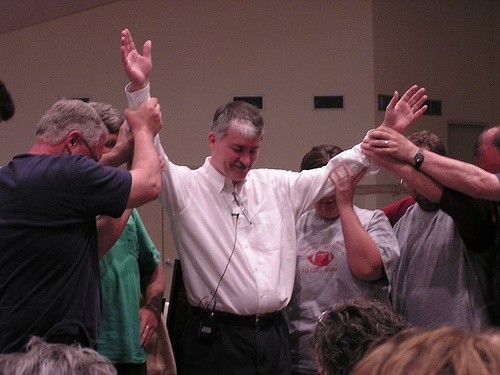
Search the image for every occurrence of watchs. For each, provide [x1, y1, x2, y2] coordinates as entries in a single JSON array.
[[140, 299, 162, 320], [414, 147, 424, 170]]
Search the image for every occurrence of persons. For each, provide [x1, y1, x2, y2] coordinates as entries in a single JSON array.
[[308, 298, 499, 375], [281, 145, 400, 375], [361, 123, 499, 334], [120, 28, 428, 374], [0, 97, 163, 354], [88, 102, 166, 375]]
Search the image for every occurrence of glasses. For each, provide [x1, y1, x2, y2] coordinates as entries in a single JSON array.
[[82, 138, 99, 163]]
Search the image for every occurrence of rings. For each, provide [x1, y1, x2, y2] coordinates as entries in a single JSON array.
[[146, 325, 151, 331], [384, 140, 388, 146]]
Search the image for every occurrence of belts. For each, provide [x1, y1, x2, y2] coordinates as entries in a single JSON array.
[[188, 305, 285, 324]]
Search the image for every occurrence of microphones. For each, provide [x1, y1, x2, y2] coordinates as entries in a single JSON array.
[[231, 213, 239, 218]]
